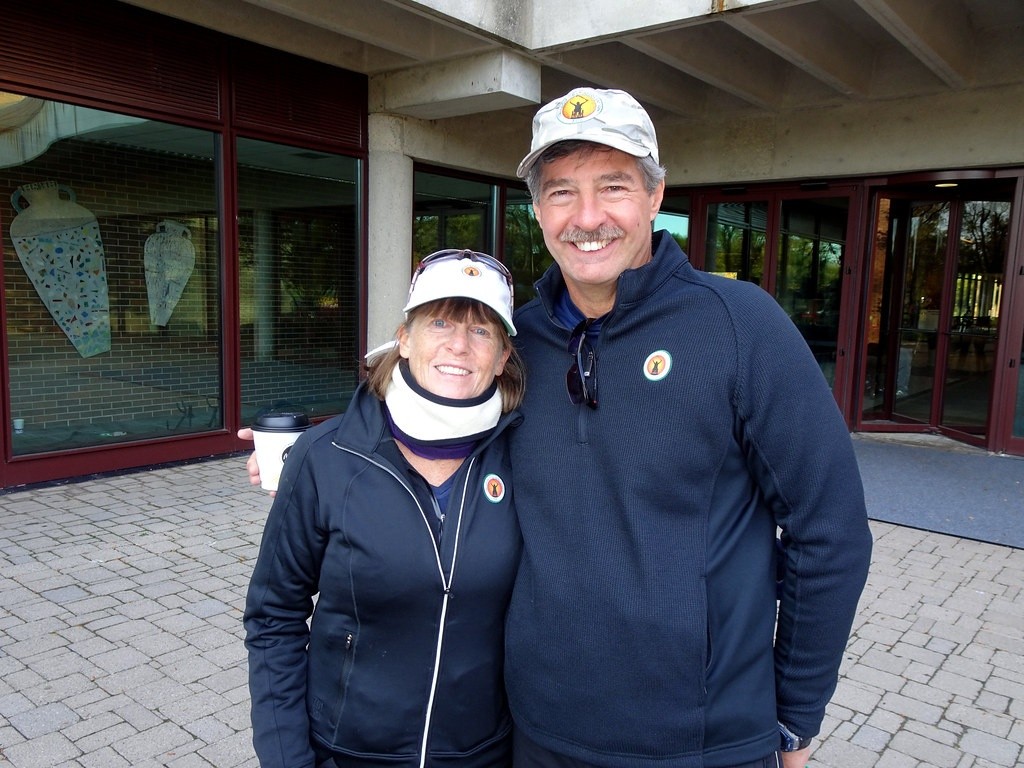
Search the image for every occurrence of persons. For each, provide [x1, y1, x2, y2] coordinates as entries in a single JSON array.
[[238, 87, 873, 768], [243, 249, 526, 768]]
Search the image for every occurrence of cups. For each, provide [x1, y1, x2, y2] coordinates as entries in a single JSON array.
[[253, 413, 313, 492], [14, 419, 24, 433]]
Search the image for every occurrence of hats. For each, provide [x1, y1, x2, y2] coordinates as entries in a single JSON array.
[[403, 258, 518, 336], [516, 88, 658, 178]]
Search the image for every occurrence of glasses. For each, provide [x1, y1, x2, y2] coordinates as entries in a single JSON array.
[[408, 249, 514, 319], [565, 318, 597, 410]]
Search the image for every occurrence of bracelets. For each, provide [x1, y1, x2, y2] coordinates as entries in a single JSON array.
[[778, 721, 812, 752]]
